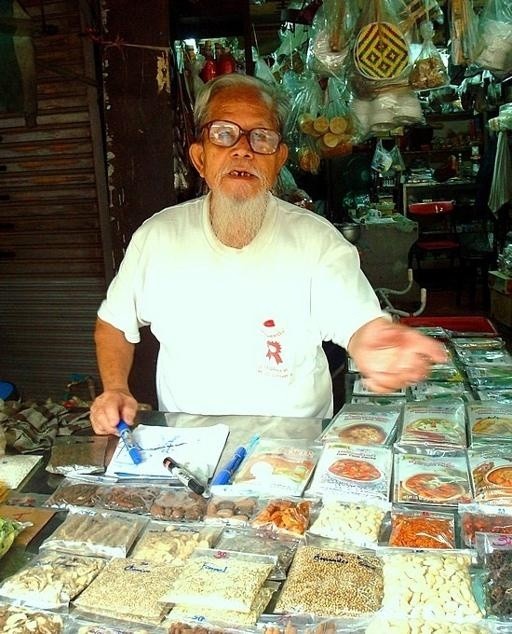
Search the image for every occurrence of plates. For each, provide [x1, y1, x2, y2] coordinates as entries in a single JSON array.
[[328, 421, 512, 506]]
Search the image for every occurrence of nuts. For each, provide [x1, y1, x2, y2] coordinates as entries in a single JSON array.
[[310, 502, 491, 634], [150, 493, 257, 524]]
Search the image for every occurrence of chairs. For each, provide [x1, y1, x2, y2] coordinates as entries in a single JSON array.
[[408, 199, 464, 305]]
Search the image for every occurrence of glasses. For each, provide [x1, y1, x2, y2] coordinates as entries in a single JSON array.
[[195, 118, 283, 156]]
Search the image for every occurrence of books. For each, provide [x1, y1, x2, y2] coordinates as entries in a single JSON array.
[[104, 422, 233, 487]]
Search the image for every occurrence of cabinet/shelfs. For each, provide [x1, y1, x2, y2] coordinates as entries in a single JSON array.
[[396, 110, 498, 289]]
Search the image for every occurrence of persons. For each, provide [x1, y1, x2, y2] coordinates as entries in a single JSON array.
[[86, 71, 452, 439]]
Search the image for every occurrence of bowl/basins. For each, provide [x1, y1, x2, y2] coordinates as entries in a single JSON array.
[[342, 225, 361, 242]]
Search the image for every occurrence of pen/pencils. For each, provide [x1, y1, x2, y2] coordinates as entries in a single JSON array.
[[163, 458, 204, 496], [211, 446, 246, 484], [116, 419, 142, 464]]
